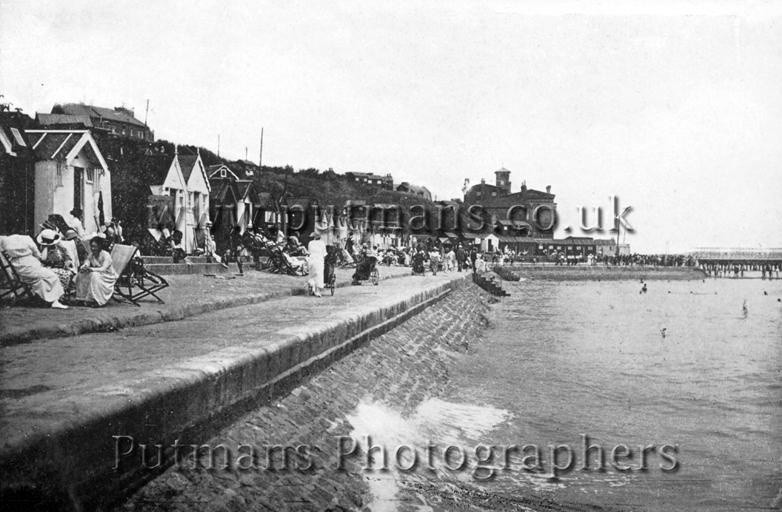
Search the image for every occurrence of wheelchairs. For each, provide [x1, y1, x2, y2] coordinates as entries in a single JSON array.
[[323, 260, 339, 295], [352, 252, 380, 287]]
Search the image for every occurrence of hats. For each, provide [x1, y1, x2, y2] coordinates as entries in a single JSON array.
[[65, 229, 77, 240], [37, 229, 61, 245]]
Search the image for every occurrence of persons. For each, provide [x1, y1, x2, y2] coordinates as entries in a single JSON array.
[[1, 200, 782, 311]]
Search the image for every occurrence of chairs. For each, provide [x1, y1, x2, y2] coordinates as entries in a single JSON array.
[[108, 243, 169, 308], [0, 249, 32, 304], [265, 240, 308, 277]]
